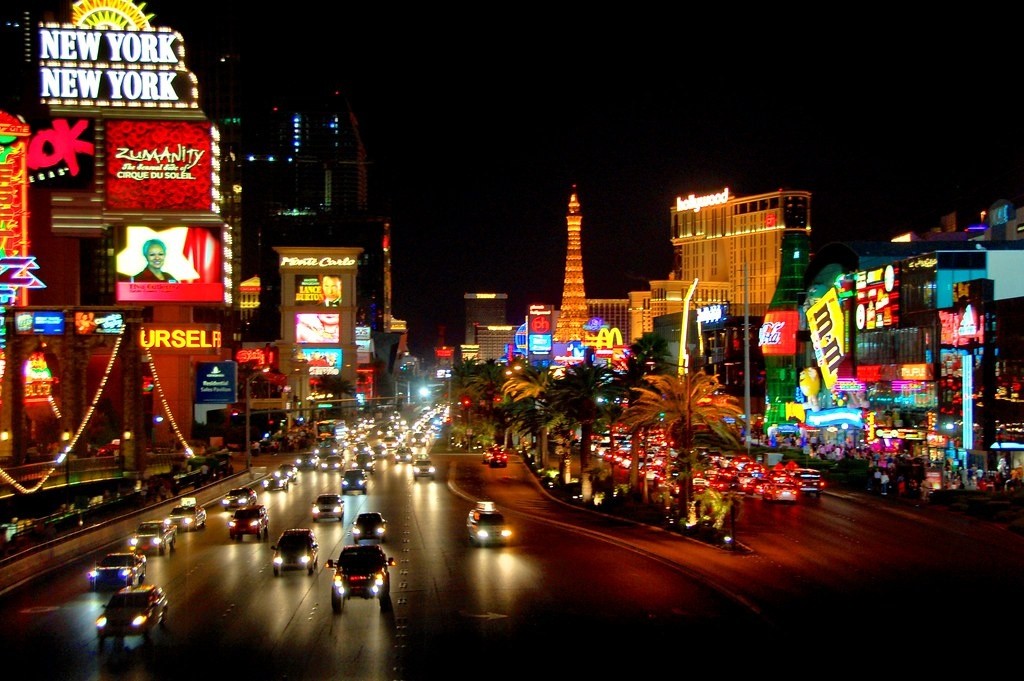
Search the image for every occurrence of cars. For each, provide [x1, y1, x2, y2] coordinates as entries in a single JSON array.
[[590, 428, 823, 504], [412, 457, 435, 483], [481, 445, 508, 468], [275, 463, 297, 481], [262, 472, 291, 491], [89, 551, 147, 592], [169, 504, 207, 531], [223, 488, 257, 511], [353, 512, 388, 545], [310, 492, 345, 523], [294, 404, 448, 473]]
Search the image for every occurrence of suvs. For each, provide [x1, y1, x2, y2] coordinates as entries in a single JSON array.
[[271, 528, 319, 575], [230, 505, 269, 542], [342, 468, 368, 495], [465, 501, 511, 547], [328, 544, 396, 601], [97, 585, 169, 642]]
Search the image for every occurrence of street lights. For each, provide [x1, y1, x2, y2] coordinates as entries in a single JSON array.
[[244, 367, 274, 472]]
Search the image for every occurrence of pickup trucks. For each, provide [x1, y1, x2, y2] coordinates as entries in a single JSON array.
[[134, 518, 179, 555]]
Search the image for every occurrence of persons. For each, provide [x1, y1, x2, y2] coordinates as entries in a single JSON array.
[[297, 275, 343, 342], [134, 240, 179, 284], [764, 433, 1024, 492]]
